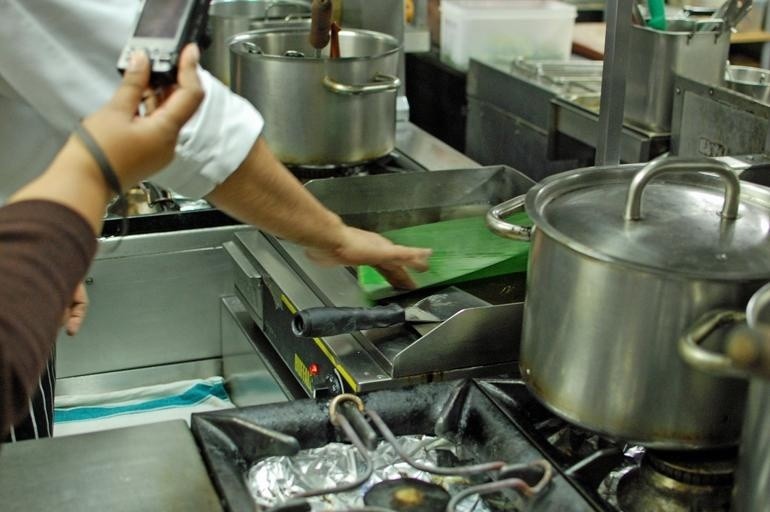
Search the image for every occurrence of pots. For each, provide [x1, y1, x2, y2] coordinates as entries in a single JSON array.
[[484, 147, 770, 454], [208, 1, 401, 168], [623, 18, 733, 130]]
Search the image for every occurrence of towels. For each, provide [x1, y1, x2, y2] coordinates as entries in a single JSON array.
[[49, 376, 237, 442]]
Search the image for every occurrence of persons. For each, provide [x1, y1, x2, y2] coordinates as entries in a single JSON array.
[[0, 1, 432, 290], [0, 43, 205, 448]]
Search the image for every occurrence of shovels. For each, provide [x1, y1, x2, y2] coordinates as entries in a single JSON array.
[[291, 285, 494, 338]]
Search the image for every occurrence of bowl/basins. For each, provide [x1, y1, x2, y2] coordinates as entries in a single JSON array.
[[672, 282, 769, 511], [725, 64, 769, 103]]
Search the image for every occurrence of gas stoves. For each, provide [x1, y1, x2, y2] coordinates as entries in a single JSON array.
[[191, 364, 770, 512], [280, 146, 429, 183]]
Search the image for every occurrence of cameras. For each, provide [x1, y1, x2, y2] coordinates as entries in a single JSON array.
[[114, 0, 212, 85]]
